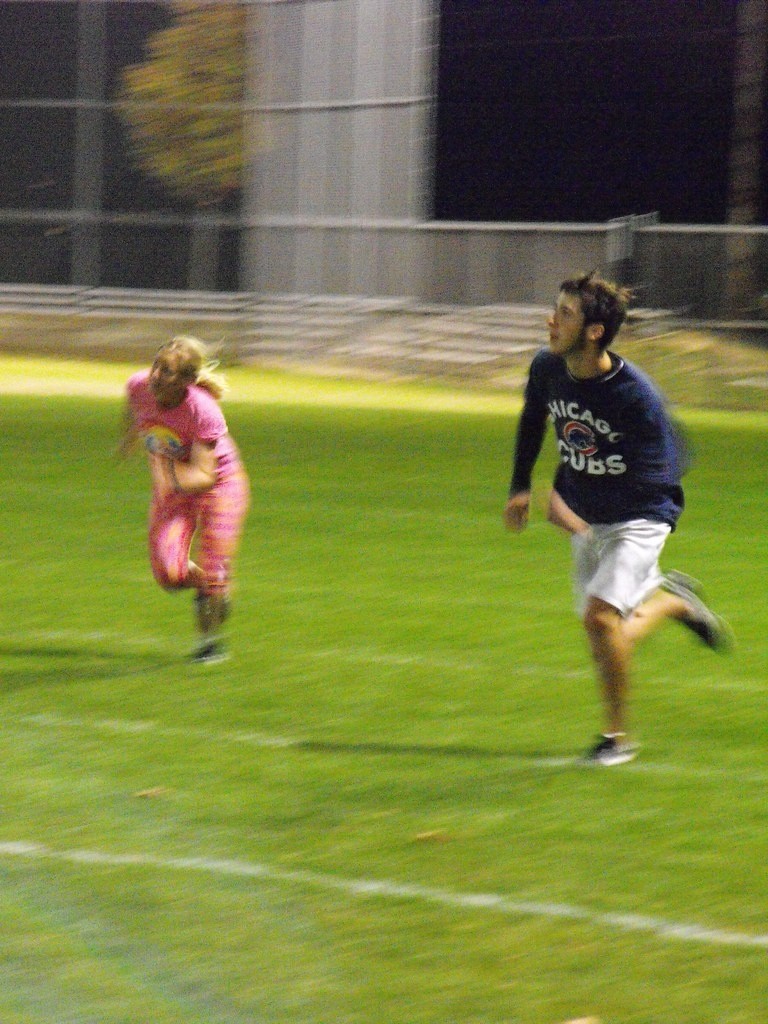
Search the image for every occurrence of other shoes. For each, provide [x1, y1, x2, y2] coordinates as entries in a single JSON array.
[[185, 638, 234, 666], [662, 571, 731, 651], [579, 724, 648, 771], [197, 587, 229, 635]]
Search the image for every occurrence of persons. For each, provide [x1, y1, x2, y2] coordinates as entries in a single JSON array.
[[503, 271, 737, 768], [110, 335, 249, 666]]
